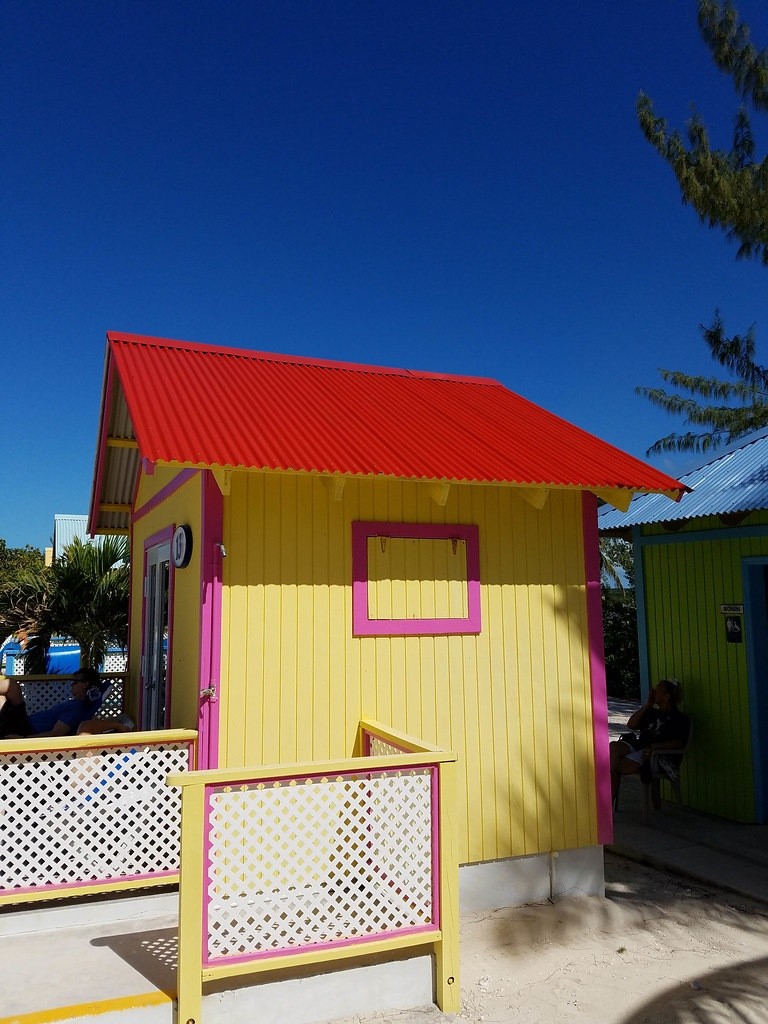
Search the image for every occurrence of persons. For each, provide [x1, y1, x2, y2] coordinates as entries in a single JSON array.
[[0, 669, 130, 814], [609, 680, 690, 800]]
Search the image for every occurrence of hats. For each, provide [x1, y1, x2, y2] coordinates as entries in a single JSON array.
[[72, 668, 100, 681], [658, 680, 674, 693]]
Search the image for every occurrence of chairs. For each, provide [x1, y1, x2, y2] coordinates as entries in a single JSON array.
[[76, 681, 113, 735], [610, 712, 693, 828]]
